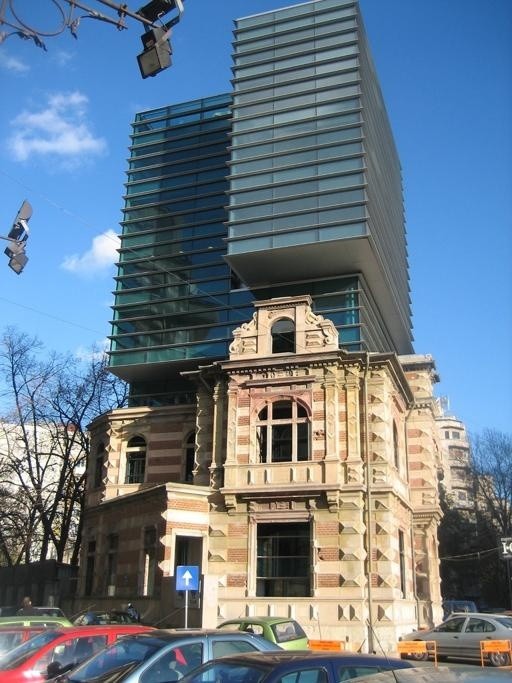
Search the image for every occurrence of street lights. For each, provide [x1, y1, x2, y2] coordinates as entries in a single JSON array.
[[0, 0, 185, 80]]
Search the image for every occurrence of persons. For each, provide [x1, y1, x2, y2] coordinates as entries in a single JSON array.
[[127, 603, 140, 622], [16, 596, 43, 616]]
